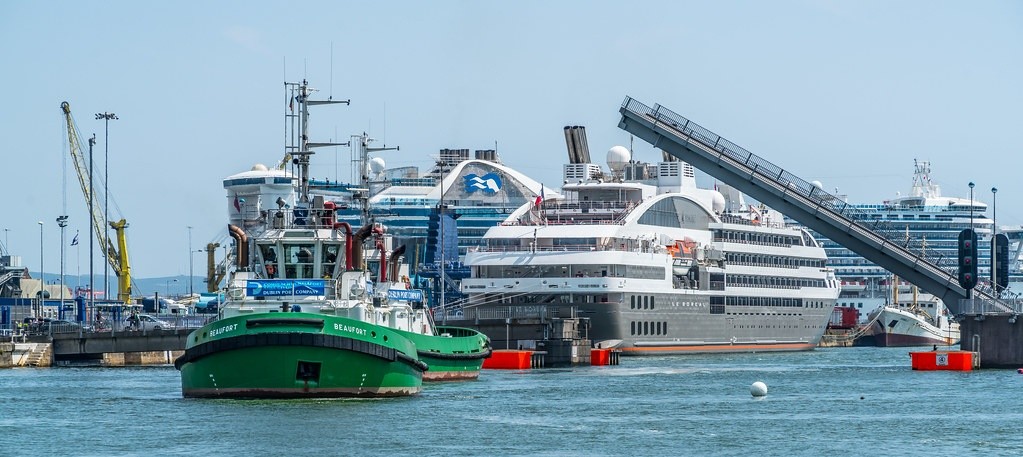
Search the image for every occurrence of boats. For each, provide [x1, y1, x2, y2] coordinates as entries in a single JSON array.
[[168, 190, 492, 407], [0, 50, 1022, 372]]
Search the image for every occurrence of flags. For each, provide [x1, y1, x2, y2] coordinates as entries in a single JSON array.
[[71, 234, 79, 246], [233, 192, 240, 212]]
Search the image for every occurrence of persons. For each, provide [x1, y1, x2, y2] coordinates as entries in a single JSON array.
[[135, 310, 141, 330], [16, 313, 45, 336], [129, 311, 137, 329], [95, 311, 107, 331]]
